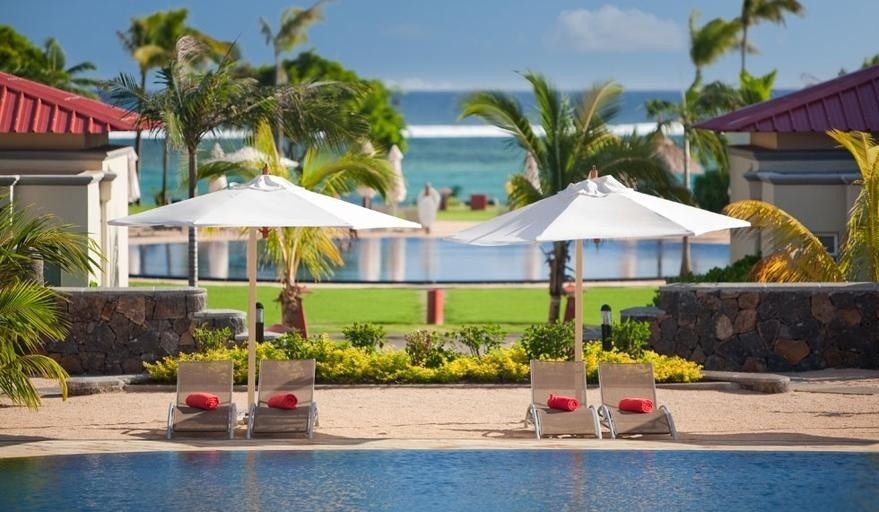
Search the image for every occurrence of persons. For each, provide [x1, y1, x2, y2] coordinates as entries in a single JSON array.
[[415, 183, 441, 234]]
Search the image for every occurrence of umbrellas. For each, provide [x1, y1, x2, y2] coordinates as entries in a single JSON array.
[[354, 138, 378, 209], [204, 139, 226, 194], [645, 132, 709, 174], [105, 171, 424, 408], [379, 144, 406, 210], [440, 166, 757, 406]]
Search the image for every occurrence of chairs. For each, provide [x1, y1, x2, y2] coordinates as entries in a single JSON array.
[[168, 355, 321, 439], [522, 356, 680, 442]]
